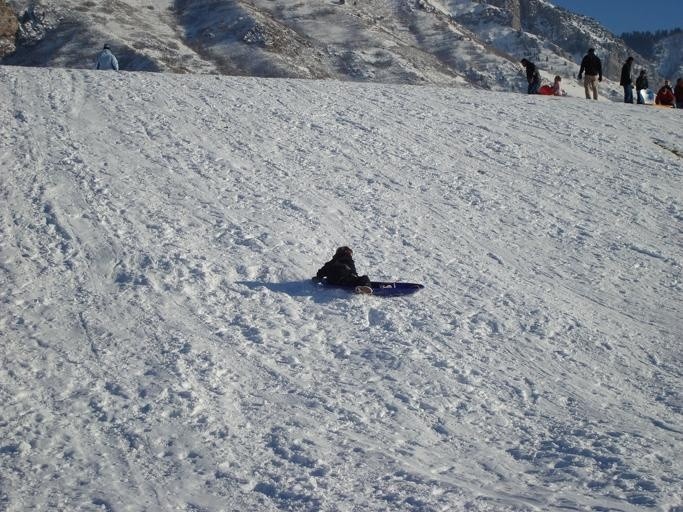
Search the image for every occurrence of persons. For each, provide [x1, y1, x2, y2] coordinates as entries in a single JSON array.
[[93, 44, 118, 72], [655, 87, 676, 109], [619, 57, 634, 104], [577, 48, 603, 100], [519, 59, 541, 94], [550, 76, 562, 96], [635, 71, 648, 104], [662, 80, 674, 95], [673, 78, 683, 109], [310, 246, 374, 295]]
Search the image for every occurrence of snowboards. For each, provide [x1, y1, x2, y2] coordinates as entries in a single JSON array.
[[319, 277, 425, 296]]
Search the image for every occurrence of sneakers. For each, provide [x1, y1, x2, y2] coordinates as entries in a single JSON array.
[[355, 286, 372, 294]]
